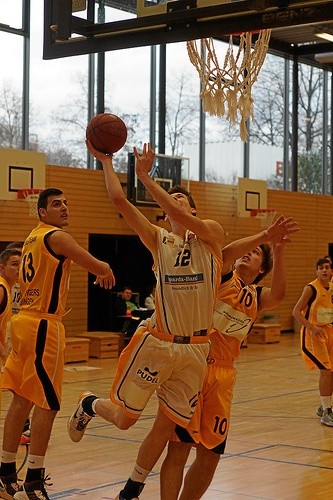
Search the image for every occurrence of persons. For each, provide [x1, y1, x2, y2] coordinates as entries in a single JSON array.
[[0, 189, 116, 500], [160, 216, 300, 500], [114, 286, 141, 334], [292, 255, 333, 426], [65, 138, 226, 500], [145, 285, 156, 309], [0, 242, 54, 446]]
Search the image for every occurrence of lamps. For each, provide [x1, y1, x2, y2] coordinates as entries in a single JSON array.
[[314, 27, 333, 42]]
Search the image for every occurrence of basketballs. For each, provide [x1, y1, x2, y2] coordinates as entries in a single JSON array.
[[86, 113, 128, 154]]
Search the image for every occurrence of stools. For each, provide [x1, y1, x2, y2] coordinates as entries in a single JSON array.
[[248, 323, 283, 344], [62, 331, 124, 364]]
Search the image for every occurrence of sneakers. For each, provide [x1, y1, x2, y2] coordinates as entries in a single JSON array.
[[320, 408, 333, 427], [66, 391, 96, 442], [18, 429, 30, 444], [315, 404, 323, 417], [0, 478, 19, 500], [114, 490, 139, 500], [12, 483, 48, 500]]
